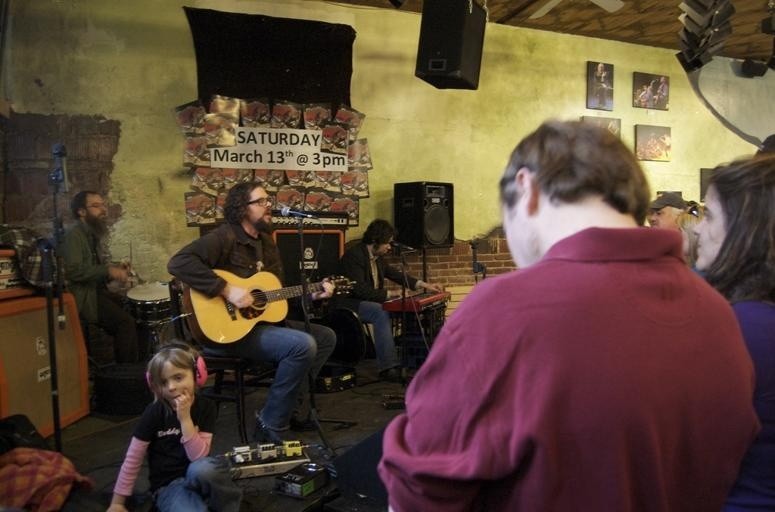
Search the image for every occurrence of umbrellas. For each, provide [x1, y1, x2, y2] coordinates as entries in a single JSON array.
[[254, 422, 296, 443]]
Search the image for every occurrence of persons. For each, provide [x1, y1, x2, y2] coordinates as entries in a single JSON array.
[[692, 153, 775, 512], [646, 190, 703, 271], [378, 118, 763, 512], [636, 133, 671, 161], [328, 218, 443, 383], [166, 181, 337, 444], [54, 191, 140, 365], [608, 119, 619, 135], [593, 63, 610, 109], [107, 339, 243, 512], [633, 76, 669, 110]]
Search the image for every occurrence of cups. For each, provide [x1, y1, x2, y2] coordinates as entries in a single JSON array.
[[247, 196, 273, 207], [82, 201, 107, 210]]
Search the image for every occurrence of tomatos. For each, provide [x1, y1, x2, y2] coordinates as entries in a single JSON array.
[[383, 290, 452, 313]]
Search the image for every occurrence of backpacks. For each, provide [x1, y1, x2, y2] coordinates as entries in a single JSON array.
[[127, 281, 176, 323]]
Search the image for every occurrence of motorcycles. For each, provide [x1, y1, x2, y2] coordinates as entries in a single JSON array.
[[295, 217, 360, 458], [357, 251, 414, 389]]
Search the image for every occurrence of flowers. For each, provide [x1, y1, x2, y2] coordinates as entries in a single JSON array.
[[53, 143, 69, 193], [390, 241, 413, 251], [280, 206, 318, 219]]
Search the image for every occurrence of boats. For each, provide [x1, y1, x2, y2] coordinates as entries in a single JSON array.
[[416, 0, 486, 89], [394, 182, 454, 250]]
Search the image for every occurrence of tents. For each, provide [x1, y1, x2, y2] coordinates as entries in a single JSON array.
[[145, 344, 208, 393]]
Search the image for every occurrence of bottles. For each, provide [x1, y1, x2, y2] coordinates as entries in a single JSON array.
[[648, 192, 688, 210]]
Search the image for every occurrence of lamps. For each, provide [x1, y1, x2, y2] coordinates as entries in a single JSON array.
[[168, 279, 316, 445]]
